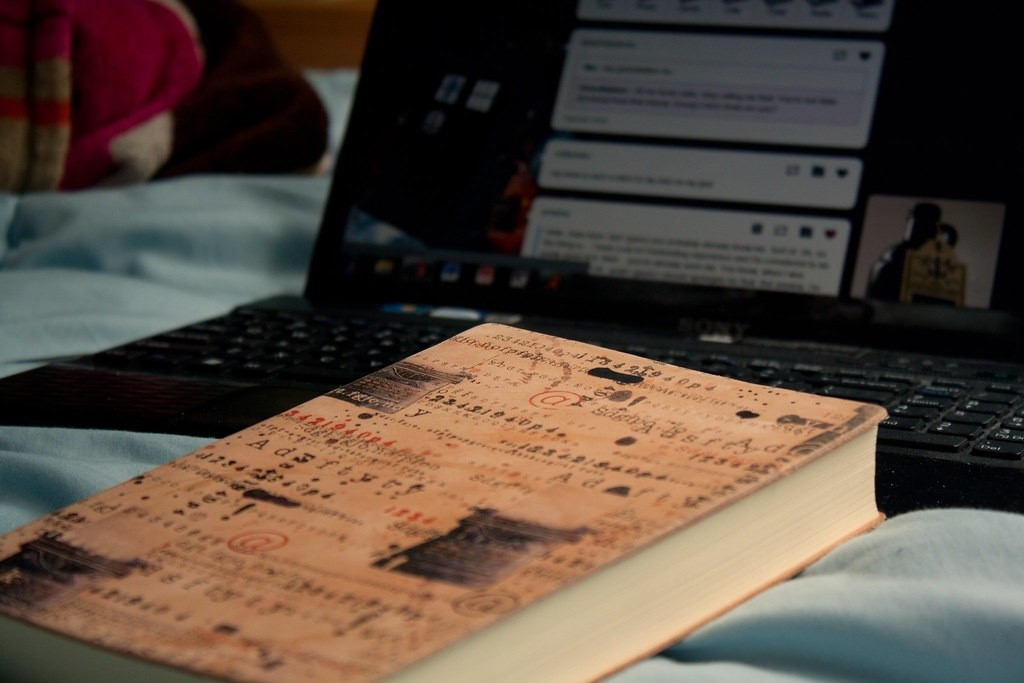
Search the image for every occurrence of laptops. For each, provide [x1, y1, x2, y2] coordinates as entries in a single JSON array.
[[1, 0, 1024, 514]]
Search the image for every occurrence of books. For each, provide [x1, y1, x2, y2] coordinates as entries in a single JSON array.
[[1, 322, 892, 683]]
